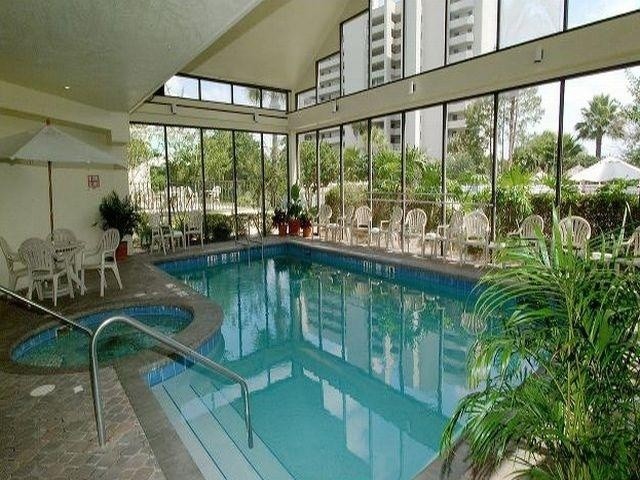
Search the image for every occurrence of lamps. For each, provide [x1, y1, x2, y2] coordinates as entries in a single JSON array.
[[0, 228, 122, 308], [145, 210, 203, 255], [310, 204, 591, 269]]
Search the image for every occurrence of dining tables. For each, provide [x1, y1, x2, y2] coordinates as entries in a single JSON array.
[[92, 191, 141, 257], [271, 180, 312, 238]]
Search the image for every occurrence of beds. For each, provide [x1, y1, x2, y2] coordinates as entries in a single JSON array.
[[1, 116, 128, 245], [530, 154, 640, 184]]
